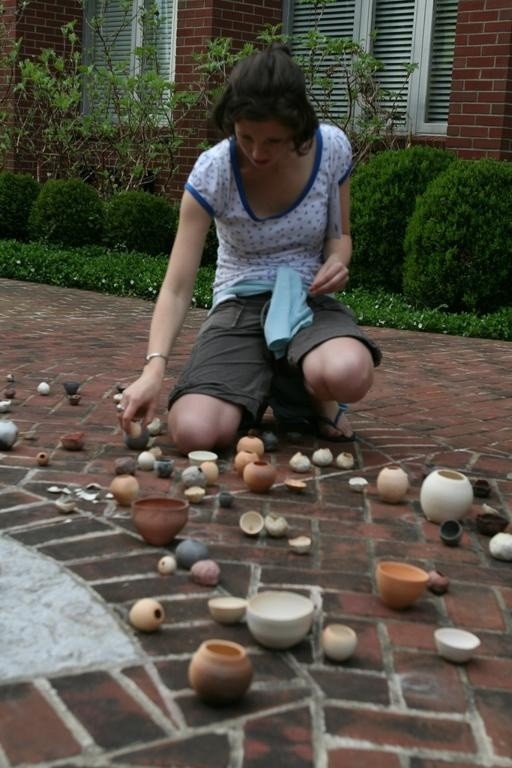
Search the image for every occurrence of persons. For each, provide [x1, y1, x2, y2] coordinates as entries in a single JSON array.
[[116, 39, 383, 454]]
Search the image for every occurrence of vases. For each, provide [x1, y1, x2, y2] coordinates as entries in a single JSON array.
[[0, 419, 18, 450], [419, 469, 475, 525], [236, 436, 265, 459], [235, 450, 261, 474], [242, 460, 276, 494], [376, 465, 409, 504], [189, 638, 254, 710]]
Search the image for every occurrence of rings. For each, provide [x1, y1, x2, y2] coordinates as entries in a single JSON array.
[[117, 404, 125, 411]]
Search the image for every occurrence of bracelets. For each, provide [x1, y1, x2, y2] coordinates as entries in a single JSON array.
[[144, 354, 170, 364]]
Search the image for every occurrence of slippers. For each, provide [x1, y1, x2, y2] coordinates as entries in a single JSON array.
[[317, 408, 355, 442]]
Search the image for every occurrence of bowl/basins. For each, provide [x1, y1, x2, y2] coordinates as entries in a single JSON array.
[[240, 511, 264, 536], [434, 628, 482, 663], [188, 449, 218, 467], [208, 596, 246, 626]]
